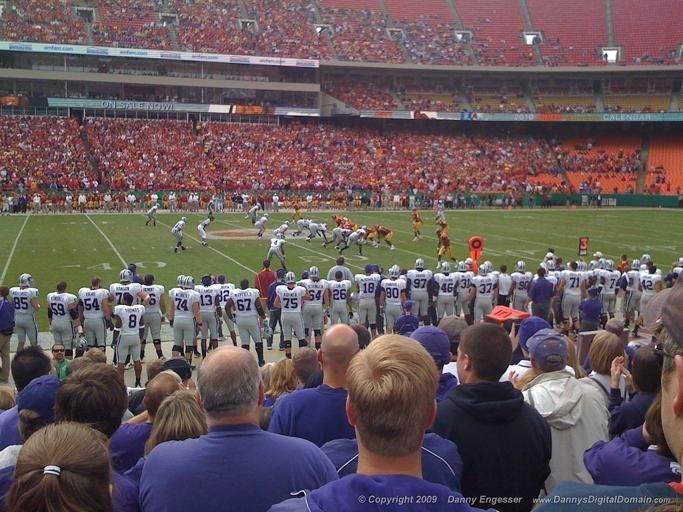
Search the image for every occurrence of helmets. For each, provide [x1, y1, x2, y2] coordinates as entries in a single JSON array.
[[262, 327, 272, 339], [678, 258, 683, 266], [278, 266, 319, 281], [19, 273, 32, 288], [362, 226, 367, 230], [182, 217, 186, 222], [277, 233, 281, 238], [442, 257, 493, 276], [285, 221, 289, 224], [546, 260, 555, 270], [176, 275, 194, 288], [73, 336, 87, 350], [589, 258, 613, 269], [119, 269, 133, 283], [264, 213, 268, 218], [515, 260, 526, 272], [631, 254, 650, 270], [388, 265, 401, 278], [416, 258, 424, 269]]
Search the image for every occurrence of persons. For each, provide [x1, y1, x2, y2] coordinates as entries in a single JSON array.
[[0, 0, 682, 512]]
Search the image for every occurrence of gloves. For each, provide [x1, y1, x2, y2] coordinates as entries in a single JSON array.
[[106, 319, 114, 330]]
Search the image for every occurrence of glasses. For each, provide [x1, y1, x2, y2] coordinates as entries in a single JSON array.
[[53, 349, 65, 352], [655, 344, 675, 365]]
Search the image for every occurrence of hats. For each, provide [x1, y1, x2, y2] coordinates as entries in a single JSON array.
[[518, 316, 568, 372], [404, 301, 412, 309], [365, 264, 378, 273], [202, 275, 225, 285], [438, 316, 468, 342], [128, 264, 136, 272], [17, 375, 62, 424], [606, 319, 624, 337], [545, 253, 554, 259], [644, 269, 683, 347], [593, 252, 602, 258], [404, 325, 450, 360]]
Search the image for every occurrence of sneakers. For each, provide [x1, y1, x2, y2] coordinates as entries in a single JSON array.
[[358, 253, 363, 256], [412, 236, 424, 241], [364, 241, 395, 250], [111, 362, 134, 370], [159, 356, 166, 361], [292, 233, 311, 242], [322, 242, 342, 254], [436, 265, 441, 269], [259, 347, 286, 367]]
